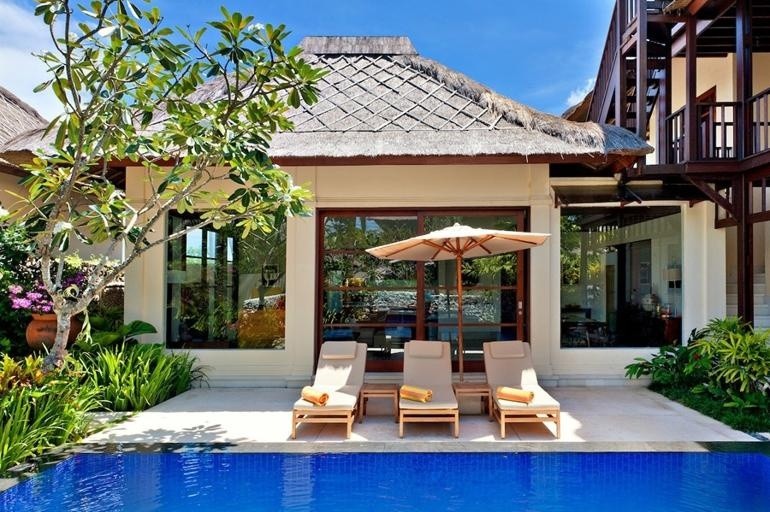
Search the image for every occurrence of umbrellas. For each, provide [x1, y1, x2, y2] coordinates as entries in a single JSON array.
[[363, 222, 552, 385]]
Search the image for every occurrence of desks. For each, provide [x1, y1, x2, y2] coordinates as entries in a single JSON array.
[[452, 383, 493, 422], [359, 383, 399, 424]]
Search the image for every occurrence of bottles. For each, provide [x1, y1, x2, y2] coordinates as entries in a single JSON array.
[[656, 303, 661, 315]]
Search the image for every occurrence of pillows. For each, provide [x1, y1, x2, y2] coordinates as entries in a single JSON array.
[[399, 385, 432, 403]]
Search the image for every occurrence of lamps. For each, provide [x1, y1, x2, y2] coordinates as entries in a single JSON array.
[[663, 269, 682, 316]]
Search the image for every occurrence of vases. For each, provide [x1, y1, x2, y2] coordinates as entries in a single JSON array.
[[26, 313, 84, 352]]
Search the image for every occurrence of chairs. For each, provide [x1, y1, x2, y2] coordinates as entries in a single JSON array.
[[483, 340, 560, 439], [399, 340, 459, 438], [292, 341, 367, 439]]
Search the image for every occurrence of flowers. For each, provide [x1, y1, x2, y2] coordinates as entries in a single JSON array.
[[8, 271, 88, 316]]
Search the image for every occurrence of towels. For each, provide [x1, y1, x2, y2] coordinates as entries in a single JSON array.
[[301, 386, 329, 406], [495, 385, 534, 404]]
